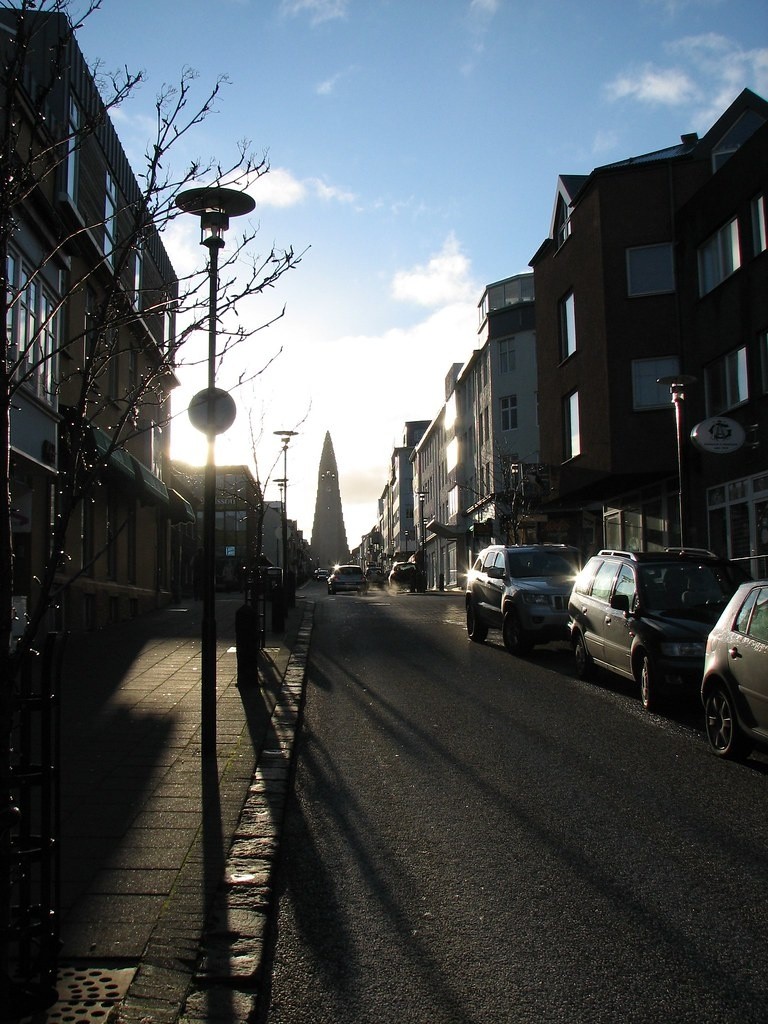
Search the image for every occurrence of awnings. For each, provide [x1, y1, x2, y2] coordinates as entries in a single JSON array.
[[57, 403, 197, 523]]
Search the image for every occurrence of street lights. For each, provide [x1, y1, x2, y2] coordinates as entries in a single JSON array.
[[417, 492, 429, 593], [657, 375, 693, 548], [423, 518, 428, 574], [273, 430, 300, 620], [404, 530, 409, 551], [274, 478, 289, 519], [175, 186, 257, 828]]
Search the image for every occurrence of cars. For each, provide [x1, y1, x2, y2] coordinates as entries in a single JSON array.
[[699, 581, 767, 761], [388, 561, 422, 592], [364, 567, 385, 586], [317, 569, 329, 581], [327, 565, 368, 595]]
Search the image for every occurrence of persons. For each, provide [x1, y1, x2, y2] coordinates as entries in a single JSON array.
[[223, 561, 234, 593]]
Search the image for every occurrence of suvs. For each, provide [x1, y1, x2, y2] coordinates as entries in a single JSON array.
[[565, 549, 754, 711], [465, 544, 581, 655]]
